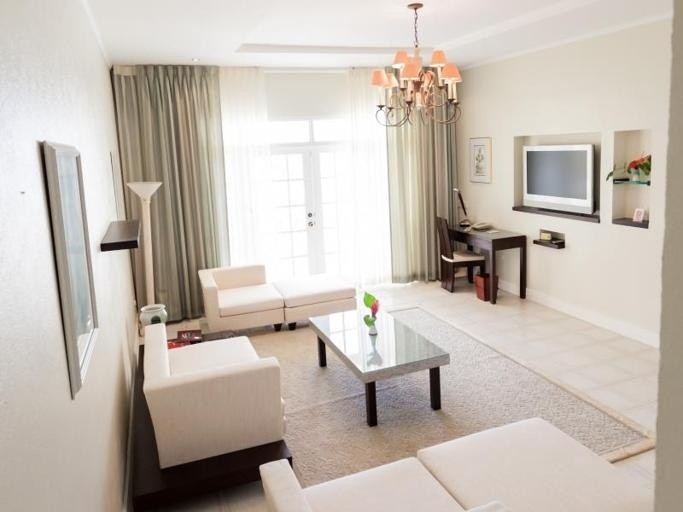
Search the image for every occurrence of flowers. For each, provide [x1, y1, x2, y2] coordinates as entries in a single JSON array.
[[606, 151, 651, 183], [363, 291, 380, 327]]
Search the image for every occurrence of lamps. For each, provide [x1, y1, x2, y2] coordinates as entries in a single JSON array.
[[452, 187, 471, 228], [126, 181, 163, 306], [372, 3, 462, 127]]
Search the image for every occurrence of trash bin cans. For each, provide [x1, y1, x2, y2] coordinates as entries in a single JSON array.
[[475, 272, 499, 301]]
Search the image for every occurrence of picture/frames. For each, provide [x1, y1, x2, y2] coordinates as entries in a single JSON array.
[[632, 208, 645, 223], [468, 137, 492, 184], [43, 141, 100, 400]]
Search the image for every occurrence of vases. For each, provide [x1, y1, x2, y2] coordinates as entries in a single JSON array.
[[369, 325, 378, 336], [138, 304, 168, 339], [632, 169, 640, 183]]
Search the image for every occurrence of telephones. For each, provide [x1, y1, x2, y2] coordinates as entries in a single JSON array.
[[472, 221, 492, 230]]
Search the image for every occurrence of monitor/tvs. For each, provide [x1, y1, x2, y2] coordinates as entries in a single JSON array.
[[522, 144, 594, 215]]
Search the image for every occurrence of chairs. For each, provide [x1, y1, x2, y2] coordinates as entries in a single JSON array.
[[141, 321, 283, 475], [259, 417, 502, 512], [198, 263, 283, 334], [434, 216, 485, 293]]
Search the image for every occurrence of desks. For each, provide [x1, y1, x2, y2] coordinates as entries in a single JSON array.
[[447, 223, 526, 304]]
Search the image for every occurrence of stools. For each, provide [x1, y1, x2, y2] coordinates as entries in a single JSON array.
[[272, 273, 356, 330], [415, 415, 655, 511]]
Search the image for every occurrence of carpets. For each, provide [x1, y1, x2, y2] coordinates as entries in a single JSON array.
[[203, 306, 656, 490]]
